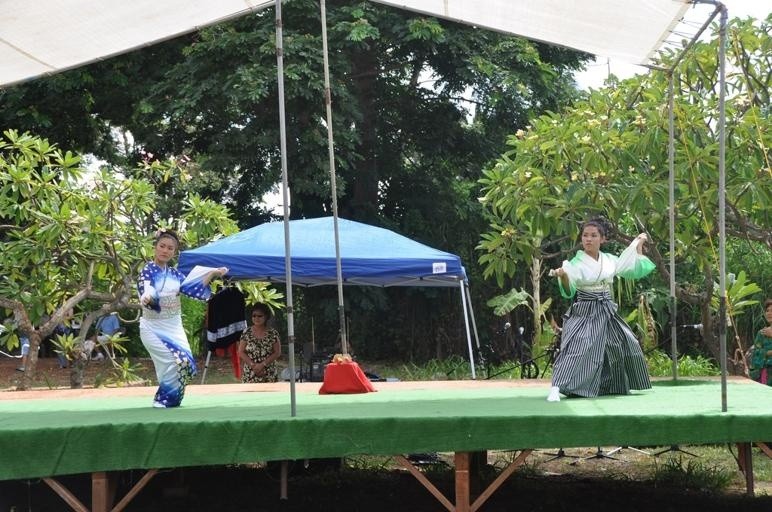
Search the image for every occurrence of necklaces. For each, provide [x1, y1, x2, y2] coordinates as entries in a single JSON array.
[[252, 325, 266, 337]]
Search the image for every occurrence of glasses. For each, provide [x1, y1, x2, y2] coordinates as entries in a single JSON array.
[[252, 314, 263, 318]]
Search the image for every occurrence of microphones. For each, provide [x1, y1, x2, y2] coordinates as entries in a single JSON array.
[[519, 327, 525, 335], [495, 321, 511, 340], [680, 323, 703, 331]]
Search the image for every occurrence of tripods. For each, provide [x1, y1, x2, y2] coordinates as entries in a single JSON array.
[[447, 332, 699, 464]]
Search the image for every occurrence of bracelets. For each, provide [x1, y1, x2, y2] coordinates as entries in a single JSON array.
[[262, 361, 268, 367]]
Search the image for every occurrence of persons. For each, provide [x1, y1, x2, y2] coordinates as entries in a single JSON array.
[[135, 231, 228, 409], [51, 302, 74, 369], [546, 219, 657, 401], [238, 302, 282, 384], [751, 299, 772, 386], [89, 305, 121, 360], [14, 288, 34, 372]]
